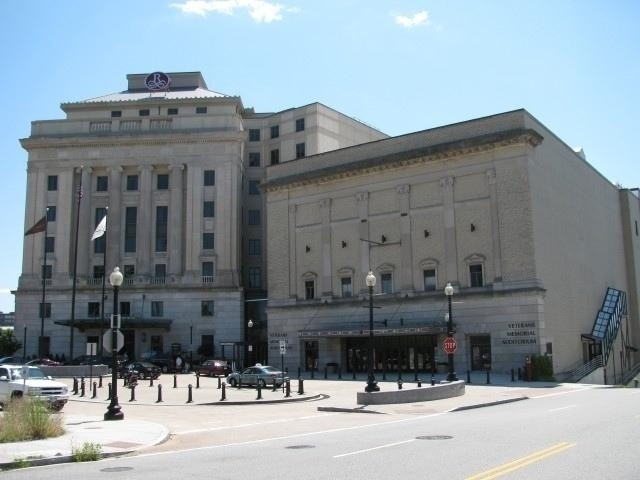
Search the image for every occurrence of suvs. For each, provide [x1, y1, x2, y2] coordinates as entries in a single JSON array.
[[0, 364, 70, 415]]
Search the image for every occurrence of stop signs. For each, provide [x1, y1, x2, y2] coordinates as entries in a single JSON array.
[[442, 337, 458, 354]]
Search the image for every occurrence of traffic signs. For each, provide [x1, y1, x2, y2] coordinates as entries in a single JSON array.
[[279, 341, 286, 355]]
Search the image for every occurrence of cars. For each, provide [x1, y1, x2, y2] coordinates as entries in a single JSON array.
[[194, 358, 229, 378], [0, 352, 186, 380], [226, 362, 290, 389]]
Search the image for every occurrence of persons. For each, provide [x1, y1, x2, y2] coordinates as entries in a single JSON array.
[[124, 350, 129, 361], [479, 351, 485, 371]]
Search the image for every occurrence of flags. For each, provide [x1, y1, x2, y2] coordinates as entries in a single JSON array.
[[25, 216, 45, 235], [91, 215, 106, 241]]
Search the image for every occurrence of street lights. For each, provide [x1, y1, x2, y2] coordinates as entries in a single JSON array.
[[22, 324, 28, 358], [445, 312, 450, 335], [364, 268, 382, 392], [445, 282, 460, 381], [103, 263, 128, 422], [247, 318, 256, 366]]
